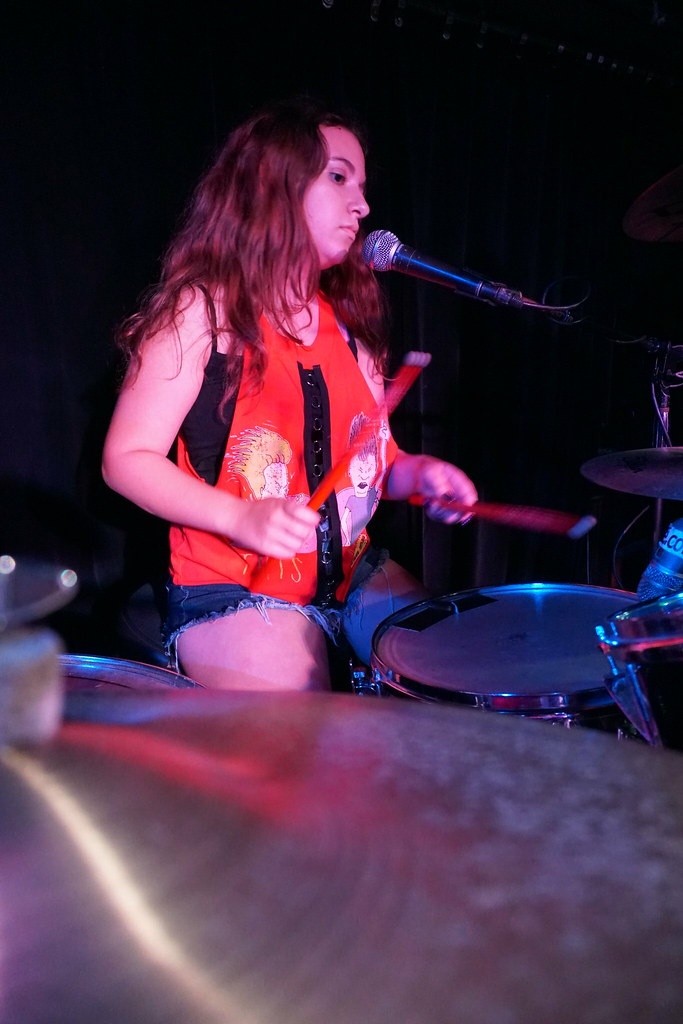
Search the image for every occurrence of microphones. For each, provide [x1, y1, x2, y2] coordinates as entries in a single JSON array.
[[361, 229, 523, 307]]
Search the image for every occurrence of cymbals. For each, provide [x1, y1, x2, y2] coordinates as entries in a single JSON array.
[[621, 166, 683, 244], [580, 446, 683, 501], [0, 688, 683, 1024]]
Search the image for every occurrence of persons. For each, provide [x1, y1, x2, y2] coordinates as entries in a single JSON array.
[[100, 105, 477, 691]]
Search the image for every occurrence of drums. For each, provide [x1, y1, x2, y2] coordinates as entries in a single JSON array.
[[57, 648, 214, 716], [372, 583, 638, 745], [595, 593, 682, 750]]
